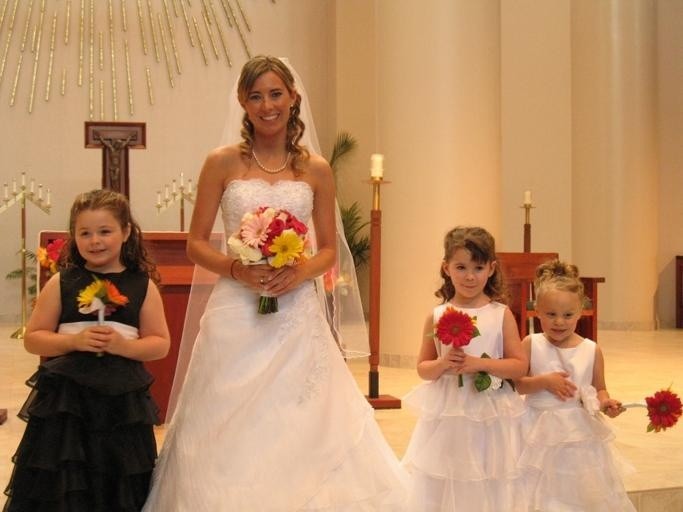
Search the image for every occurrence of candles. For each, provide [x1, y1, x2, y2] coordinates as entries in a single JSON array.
[[524, 190, 532, 204], [370, 154, 383, 177], [157, 172, 192, 204], [4, 172, 50, 203]]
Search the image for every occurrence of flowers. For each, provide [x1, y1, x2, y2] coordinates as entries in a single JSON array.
[[77, 279, 128, 358], [603, 387, 683, 433], [39, 237, 66, 275], [426, 307, 481, 388], [229, 208, 309, 314], [473, 353, 515, 392], [580, 387, 601, 416]]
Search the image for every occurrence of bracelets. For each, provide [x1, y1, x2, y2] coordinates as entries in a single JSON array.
[[230, 258, 237, 281]]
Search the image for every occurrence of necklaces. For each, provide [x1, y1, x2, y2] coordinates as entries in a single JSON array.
[[250, 146, 290, 172]]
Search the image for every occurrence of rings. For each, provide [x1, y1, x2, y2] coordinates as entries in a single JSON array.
[[260, 278, 263, 284]]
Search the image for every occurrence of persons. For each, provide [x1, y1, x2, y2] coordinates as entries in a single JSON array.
[[400, 227, 528, 511], [2, 189, 171, 511], [516, 257, 638, 512], [140, 56, 404, 511]]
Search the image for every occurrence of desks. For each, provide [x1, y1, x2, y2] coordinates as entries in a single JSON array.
[[498, 252, 605, 343]]
